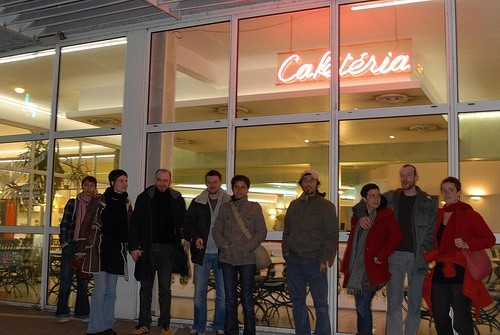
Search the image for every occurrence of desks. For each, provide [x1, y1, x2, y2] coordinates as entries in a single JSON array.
[[50, 251, 62, 258], [0, 245, 36, 251]]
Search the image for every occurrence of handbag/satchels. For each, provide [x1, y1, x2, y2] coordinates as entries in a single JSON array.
[[462, 249, 493, 284], [254, 246, 272, 269], [71, 256, 93, 278]]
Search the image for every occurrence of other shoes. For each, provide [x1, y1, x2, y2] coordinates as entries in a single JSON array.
[[87, 328, 116, 335], [57, 317, 69, 322], [74, 317, 89, 322]]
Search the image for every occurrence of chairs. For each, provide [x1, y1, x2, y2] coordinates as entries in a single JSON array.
[[401, 287, 500, 335], [206, 263, 314, 328], [0, 237, 94, 304]]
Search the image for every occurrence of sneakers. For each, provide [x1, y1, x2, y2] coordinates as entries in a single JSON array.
[[160, 326, 174, 335], [131, 323, 150, 335]]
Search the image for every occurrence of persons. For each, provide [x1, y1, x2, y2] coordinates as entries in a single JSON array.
[[75, 169, 145, 335], [55, 176, 97, 323], [425, 177, 496, 335], [352, 164, 438, 335], [187, 170, 232, 335], [127, 169, 189, 335], [212, 175, 267, 335], [340, 184, 403, 335], [281, 170, 339, 335]]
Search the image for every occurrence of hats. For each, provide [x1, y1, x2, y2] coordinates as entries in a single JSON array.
[[301, 170, 319, 179]]
